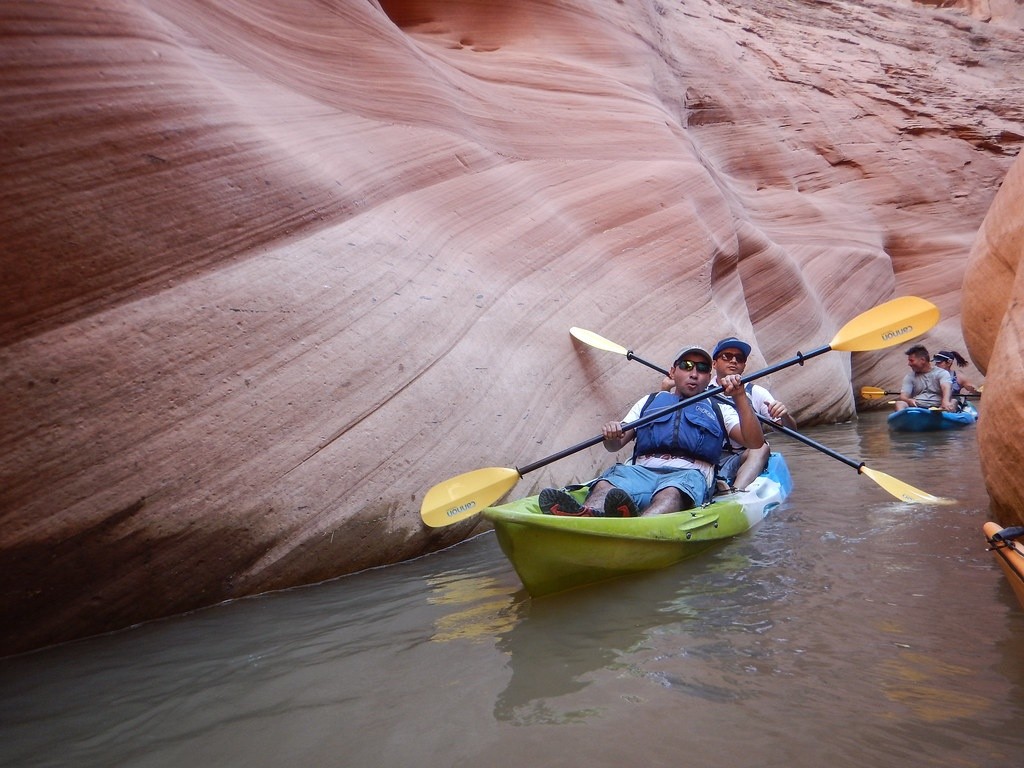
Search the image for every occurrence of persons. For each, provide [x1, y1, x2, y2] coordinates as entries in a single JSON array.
[[929, 349, 986, 412], [539, 344, 765, 519], [660, 338, 797, 493], [894, 344, 961, 413]]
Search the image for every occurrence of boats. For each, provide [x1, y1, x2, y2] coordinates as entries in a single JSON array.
[[481, 452, 795, 597], [888, 401, 980, 431]]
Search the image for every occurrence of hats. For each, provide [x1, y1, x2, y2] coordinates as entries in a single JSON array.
[[712, 337, 752, 361], [674, 345, 712, 371], [929, 354, 954, 364]]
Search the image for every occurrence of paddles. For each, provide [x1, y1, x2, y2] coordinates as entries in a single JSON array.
[[420, 295, 941, 528], [569, 327, 954, 506], [860, 386, 982, 400]]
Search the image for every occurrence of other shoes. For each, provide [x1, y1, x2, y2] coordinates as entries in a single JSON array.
[[604, 489, 639, 517], [538, 488, 602, 517], [716, 480, 732, 495]]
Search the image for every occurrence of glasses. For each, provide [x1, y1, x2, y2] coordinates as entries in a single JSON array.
[[716, 352, 746, 363], [675, 360, 711, 373]]
